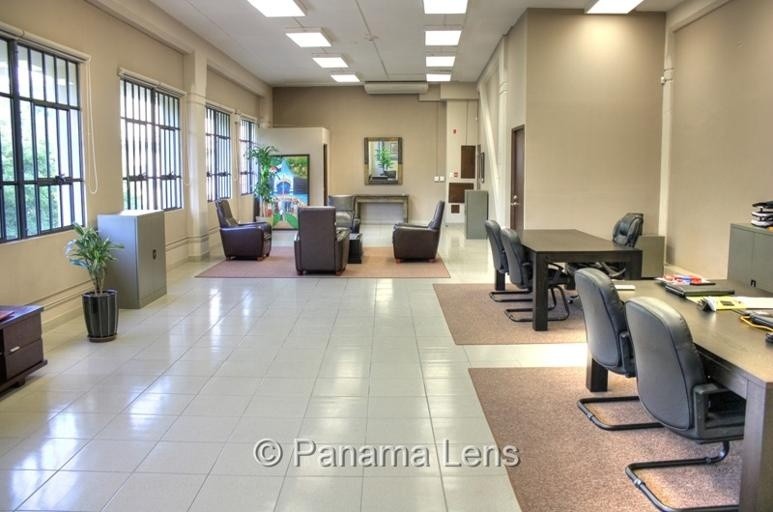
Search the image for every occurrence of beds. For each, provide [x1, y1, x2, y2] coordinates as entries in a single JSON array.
[[348, 233, 364, 264]]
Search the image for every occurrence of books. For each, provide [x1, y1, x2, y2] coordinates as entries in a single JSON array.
[[654, 274, 773, 328], [0, 312, 14, 320]]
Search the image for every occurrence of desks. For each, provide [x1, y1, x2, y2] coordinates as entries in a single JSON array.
[[611, 279, 773, 511], [352, 194, 408, 223], [514, 229, 643, 330]]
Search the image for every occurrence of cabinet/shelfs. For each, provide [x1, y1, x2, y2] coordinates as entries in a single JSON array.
[[633, 234, 664, 279], [464, 190, 488, 239], [97, 210, 167, 309], [1, 304, 47, 394], [728, 221, 773, 293]]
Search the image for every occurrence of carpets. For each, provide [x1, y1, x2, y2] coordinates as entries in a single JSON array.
[[432, 283, 587, 345], [195, 247, 450, 279], [469, 366, 745, 511]]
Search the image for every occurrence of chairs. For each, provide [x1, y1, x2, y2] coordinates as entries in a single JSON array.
[[499, 229, 569, 322], [372, 171, 396, 181], [215, 197, 272, 262], [624, 295, 746, 512], [485, 220, 533, 302], [564, 213, 643, 303], [574, 268, 665, 431], [294, 206, 350, 276], [328, 195, 361, 233], [393, 200, 444, 262]]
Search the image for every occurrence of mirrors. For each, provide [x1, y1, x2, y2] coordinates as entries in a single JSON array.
[[364, 137, 402, 185]]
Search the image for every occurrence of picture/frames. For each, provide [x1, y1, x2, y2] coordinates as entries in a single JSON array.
[[267, 153, 309, 230]]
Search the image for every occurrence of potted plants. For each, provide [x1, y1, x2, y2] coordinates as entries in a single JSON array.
[[67, 222, 124, 343], [246, 146, 279, 223]]
